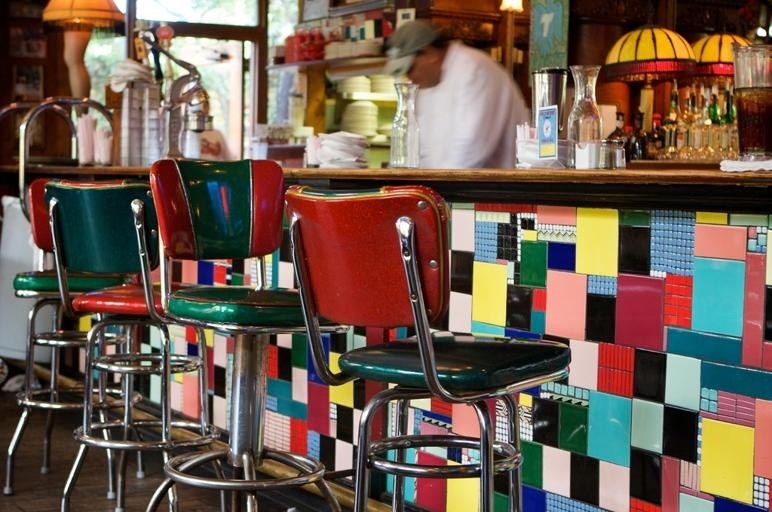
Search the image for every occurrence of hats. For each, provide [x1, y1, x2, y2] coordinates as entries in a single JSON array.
[[381, 19, 443, 77]]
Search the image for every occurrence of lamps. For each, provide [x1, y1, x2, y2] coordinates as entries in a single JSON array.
[[499, 1, 523, 14], [41, 0, 126, 31], [600, 24, 697, 131], [690, 33, 753, 95]]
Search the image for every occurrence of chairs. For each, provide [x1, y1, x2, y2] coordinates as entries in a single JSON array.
[[285, 183, 572, 512], [3, 180, 148, 499], [42, 181, 233, 511], [144, 158, 347, 512]]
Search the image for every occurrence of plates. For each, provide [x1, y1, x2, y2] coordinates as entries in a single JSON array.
[[324, 39, 395, 145]]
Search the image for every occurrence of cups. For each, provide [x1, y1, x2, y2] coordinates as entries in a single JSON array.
[[732, 42, 772, 159]]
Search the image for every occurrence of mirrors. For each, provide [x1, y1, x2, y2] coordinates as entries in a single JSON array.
[[656, 1, 772, 129]]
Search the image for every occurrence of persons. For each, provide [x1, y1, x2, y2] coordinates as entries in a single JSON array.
[[382, 18, 531, 170]]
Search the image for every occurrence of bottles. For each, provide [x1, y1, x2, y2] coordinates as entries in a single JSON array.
[[530, 67, 568, 135], [391, 81, 419, 169], [568, 65, 741, 169], [77, 114, 96, 165], [284, 25, 322, 63]]
